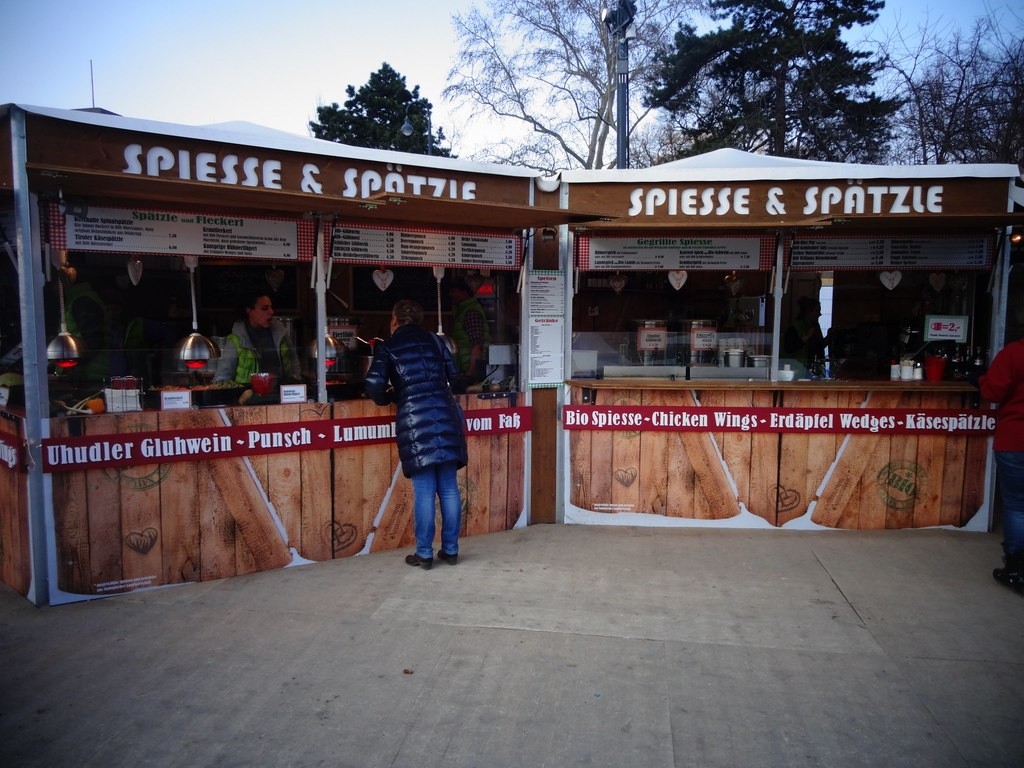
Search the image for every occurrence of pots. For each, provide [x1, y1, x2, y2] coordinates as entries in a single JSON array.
[[142, 382, 252, 408]]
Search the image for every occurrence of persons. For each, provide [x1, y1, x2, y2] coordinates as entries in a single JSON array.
[[366, 301, 467, 569], [443, 279, 492, 390], [214, 294, 301, 408], [783, 295, 836, 375], [978, 336, 1024, 597]]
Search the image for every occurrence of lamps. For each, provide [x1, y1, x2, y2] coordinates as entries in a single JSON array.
[[427, 267, 459, 354], [42, 250, 85, 368], [174, 255, 217, 369], [305, 265, 345, 364]]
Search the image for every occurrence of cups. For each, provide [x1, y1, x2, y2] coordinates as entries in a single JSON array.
[[926, 357, 946, 383], [898, 366, 913, 380], [890, 365, 900, 379], [724, 352, 745, 367], [747, 355, 770, 367]]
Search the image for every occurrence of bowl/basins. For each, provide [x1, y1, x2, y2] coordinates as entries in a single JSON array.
[[250, 373, 275, 394], [778, 370, 794, 381]]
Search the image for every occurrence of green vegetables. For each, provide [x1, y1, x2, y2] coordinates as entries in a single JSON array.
[[216, 379, 246, 388]]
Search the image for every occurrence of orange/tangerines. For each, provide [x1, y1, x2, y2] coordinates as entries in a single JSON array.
[[88, 399, 105, 413]]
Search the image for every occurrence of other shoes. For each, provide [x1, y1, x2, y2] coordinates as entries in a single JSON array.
[[993, 568, 1024, 593]]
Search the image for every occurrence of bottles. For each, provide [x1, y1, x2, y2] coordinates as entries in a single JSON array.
[[913, 362, 923, 379], [361, 339, 376, 374], [812, 355, 819, 375], [968, 347, 985, 386]]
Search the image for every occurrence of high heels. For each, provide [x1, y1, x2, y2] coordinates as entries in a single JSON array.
[[437, 550, 458, 565], [406, 553, 434, 570]]
